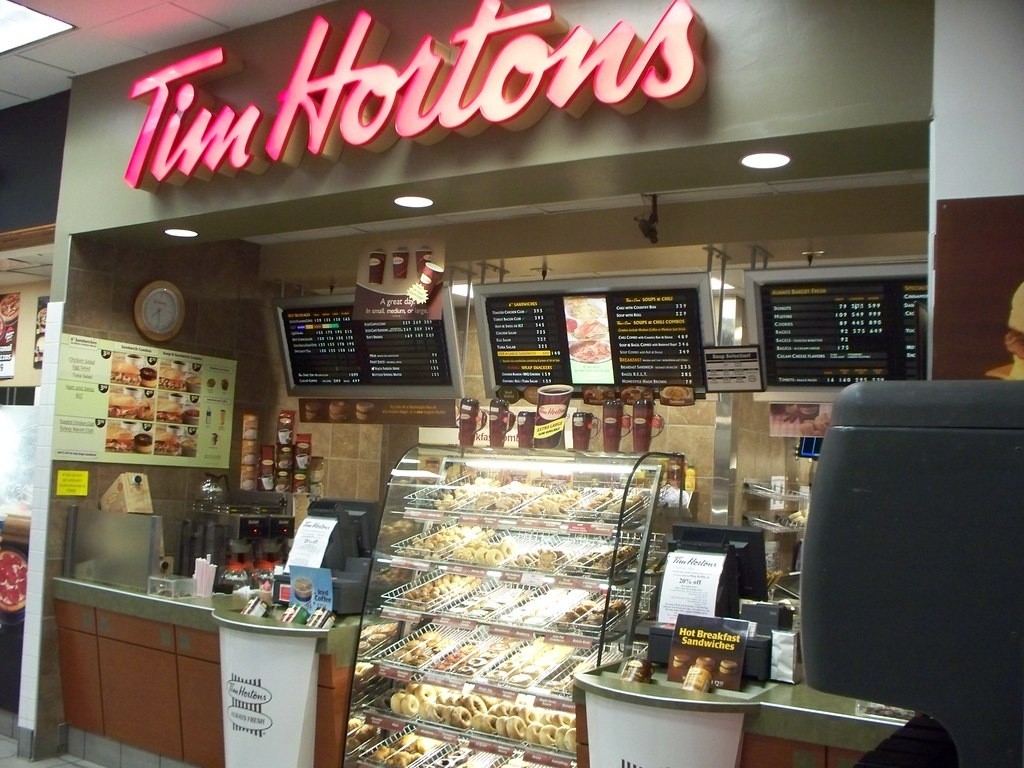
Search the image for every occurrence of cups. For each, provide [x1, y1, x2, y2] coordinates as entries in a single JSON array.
[[621, 658, 655, 684], [123, 387, 139, 398], [603, 400, 623, 453], [261, 474, 274, 491], [171, 361, 190, 373], [488, 398, 508, 448], [124, 354, 144, 367], [121, 421, 139, 433], [296, 454, 311, 470], [392, 250, 409, 279], [165, 425, 183, 436], [419, 262, 444, 298], [632, 399, 655, 452], [516, 411, 536, 450], [570, 412, 594, 452], [682, 664, 713, 693], [368, 251, 387, 286], [416, 250, 433, 277], [292, 584, 313, 611], [278, 428, 292, 445], [459, 397, 479, 446], [169, 393, 186, 405], [533, 385, 574, 449]]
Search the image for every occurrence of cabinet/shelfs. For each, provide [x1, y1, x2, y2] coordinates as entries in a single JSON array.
[[743, 481, 812, 534], [341, 442, 685, 768]]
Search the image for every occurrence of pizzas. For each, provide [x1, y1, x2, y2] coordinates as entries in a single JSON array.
[[0, 551, 28, 612], [569, 339, 611, 362], [0, 293, 21, 322], [573, 320, 601, 340]]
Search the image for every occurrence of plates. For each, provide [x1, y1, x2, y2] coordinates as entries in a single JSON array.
[[569, 339, 611, 363]]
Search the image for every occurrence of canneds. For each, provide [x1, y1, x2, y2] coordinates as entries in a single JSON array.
[[621, 657, 656, 684], [681, 664, 712, 694]]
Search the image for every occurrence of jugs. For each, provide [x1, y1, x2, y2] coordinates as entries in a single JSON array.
[[199, 471, 230, 506]]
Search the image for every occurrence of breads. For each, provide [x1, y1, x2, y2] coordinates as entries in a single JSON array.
[[788, 508, 808, 526], [396, 477, 647, 690], [344, 518, 420, 753]]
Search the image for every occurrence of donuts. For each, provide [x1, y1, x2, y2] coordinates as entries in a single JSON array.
[[673, 654, 738, 670], [306, 400, 374, 414], [388, 682, 576, 753]]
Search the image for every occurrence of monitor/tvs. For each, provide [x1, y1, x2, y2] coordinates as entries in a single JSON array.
[[308, 498, 380, 557], [665, 524, 767, 601]]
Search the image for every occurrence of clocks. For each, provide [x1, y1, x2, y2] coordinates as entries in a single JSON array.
[[134, 281, 185, 341]]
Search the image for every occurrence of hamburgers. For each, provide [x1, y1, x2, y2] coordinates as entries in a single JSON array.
[[105, 362, 188, 457]]
